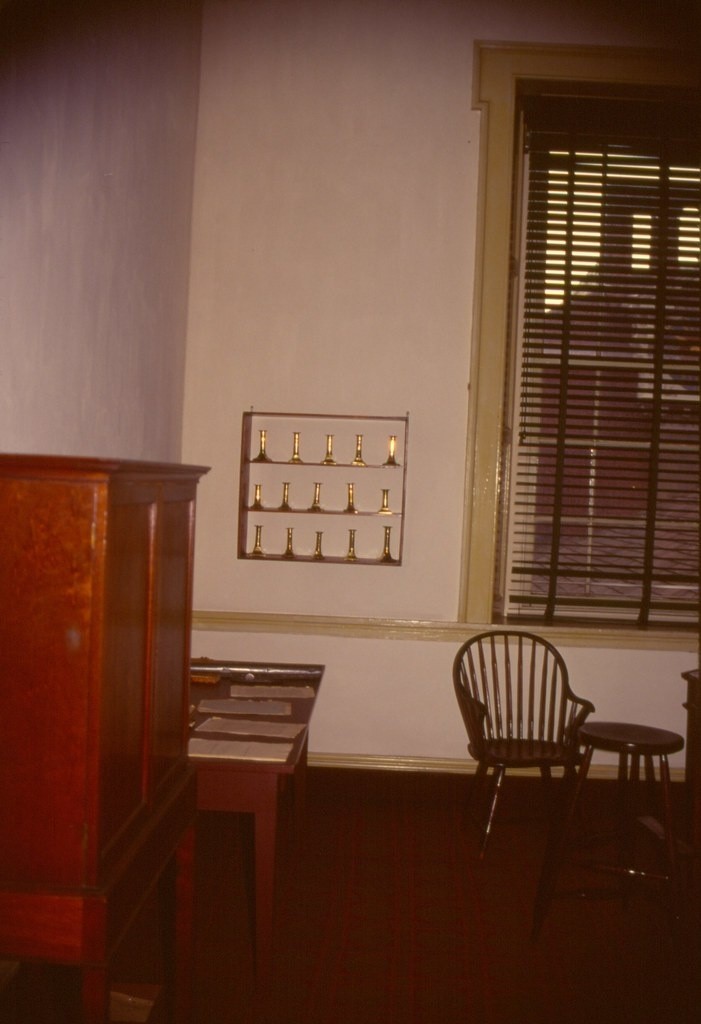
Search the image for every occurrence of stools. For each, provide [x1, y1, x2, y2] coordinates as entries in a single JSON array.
[[530, 721, 684, 949]]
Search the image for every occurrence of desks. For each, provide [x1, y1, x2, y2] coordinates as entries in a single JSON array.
[[184, 660, 326, 1003]]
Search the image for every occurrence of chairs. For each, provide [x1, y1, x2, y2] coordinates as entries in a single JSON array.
[[453, 630, 595, 865]]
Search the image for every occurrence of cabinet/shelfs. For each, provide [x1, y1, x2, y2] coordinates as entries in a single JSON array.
[[0, 453, 213, 1024], [236, 412, 409, 567]]
